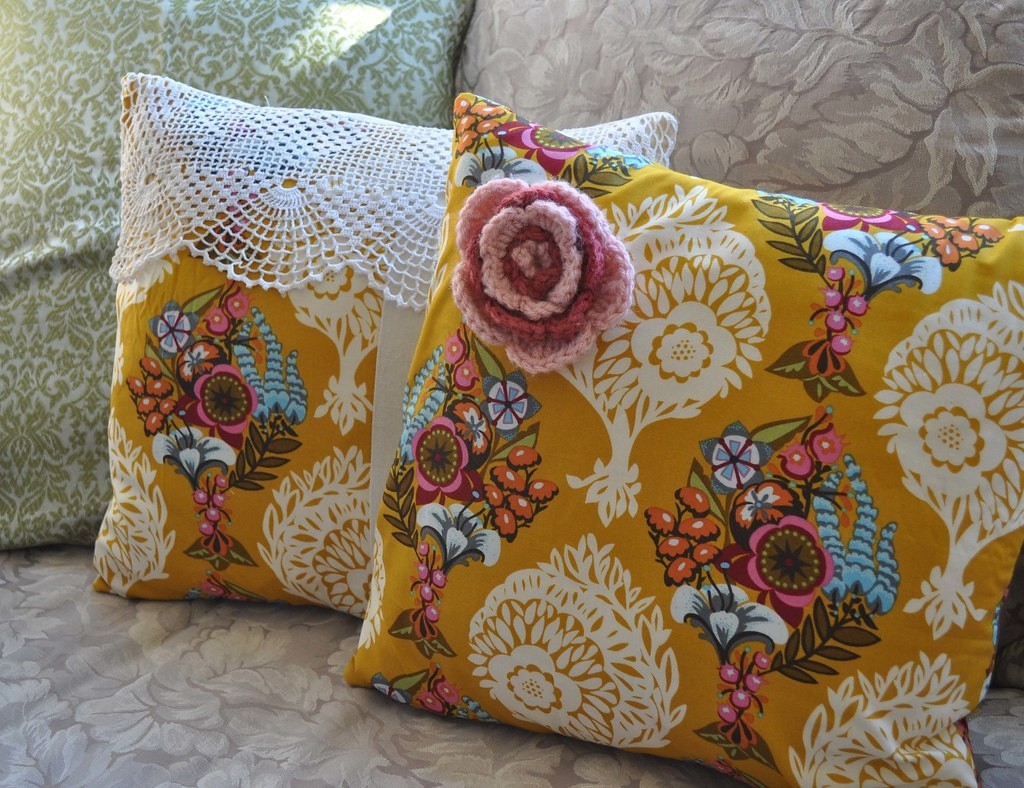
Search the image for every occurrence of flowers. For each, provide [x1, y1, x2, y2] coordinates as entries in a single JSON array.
[[451, 178, 635, 376]]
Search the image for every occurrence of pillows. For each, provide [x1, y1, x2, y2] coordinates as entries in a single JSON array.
[[455, 1, 1024, 222], [0, 0, 477, 554], [341, 92, 1023, 788], [90, 71, 677, 622]]
